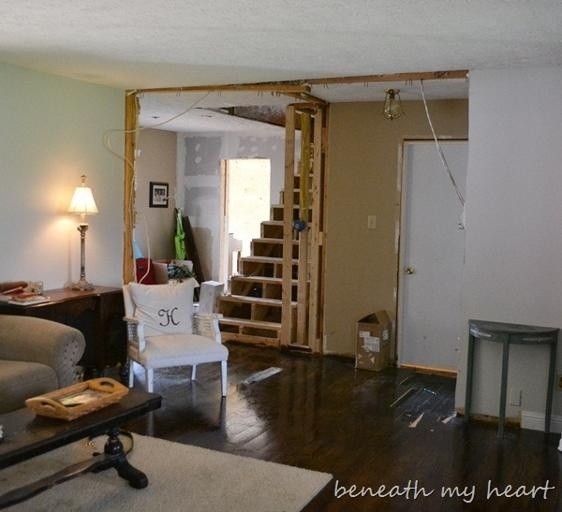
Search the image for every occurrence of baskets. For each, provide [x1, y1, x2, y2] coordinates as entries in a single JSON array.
[[26, 377, 130, 422]]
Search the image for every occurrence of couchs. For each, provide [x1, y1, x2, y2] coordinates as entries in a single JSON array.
[[0, 314, 87, 416]]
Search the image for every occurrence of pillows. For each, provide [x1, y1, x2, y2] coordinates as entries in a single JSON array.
[[128, 281, 193, 336]]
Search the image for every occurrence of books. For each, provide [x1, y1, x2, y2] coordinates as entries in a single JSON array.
[[8, 295, 51, 306]]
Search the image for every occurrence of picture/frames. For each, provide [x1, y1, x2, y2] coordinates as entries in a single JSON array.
[[149, 181, 169, 208]]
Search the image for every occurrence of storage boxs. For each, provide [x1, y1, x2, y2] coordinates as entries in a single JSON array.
[[200, 281, 224, 315], [356, 310, 391, 372]]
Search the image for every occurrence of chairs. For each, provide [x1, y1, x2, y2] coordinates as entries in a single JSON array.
[[121, 284, 230, 398], [151, 258, 199, 287]]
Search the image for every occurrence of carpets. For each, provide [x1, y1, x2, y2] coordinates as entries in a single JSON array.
[[0, 430, 334, 512]]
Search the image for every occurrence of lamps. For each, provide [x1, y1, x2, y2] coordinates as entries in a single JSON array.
[[66, 174, 99, 291], [380, 88, 405, 121]]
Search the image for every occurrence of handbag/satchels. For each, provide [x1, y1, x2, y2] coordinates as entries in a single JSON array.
[[174, 232, 188, 260]]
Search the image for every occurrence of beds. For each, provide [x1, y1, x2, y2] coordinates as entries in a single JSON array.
[[0, 391, 162, 509]]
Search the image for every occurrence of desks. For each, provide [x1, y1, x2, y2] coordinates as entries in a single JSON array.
[[228, 237, 243, 276], [463, 319, 560, 440], [0, 286, 127, 370]]
